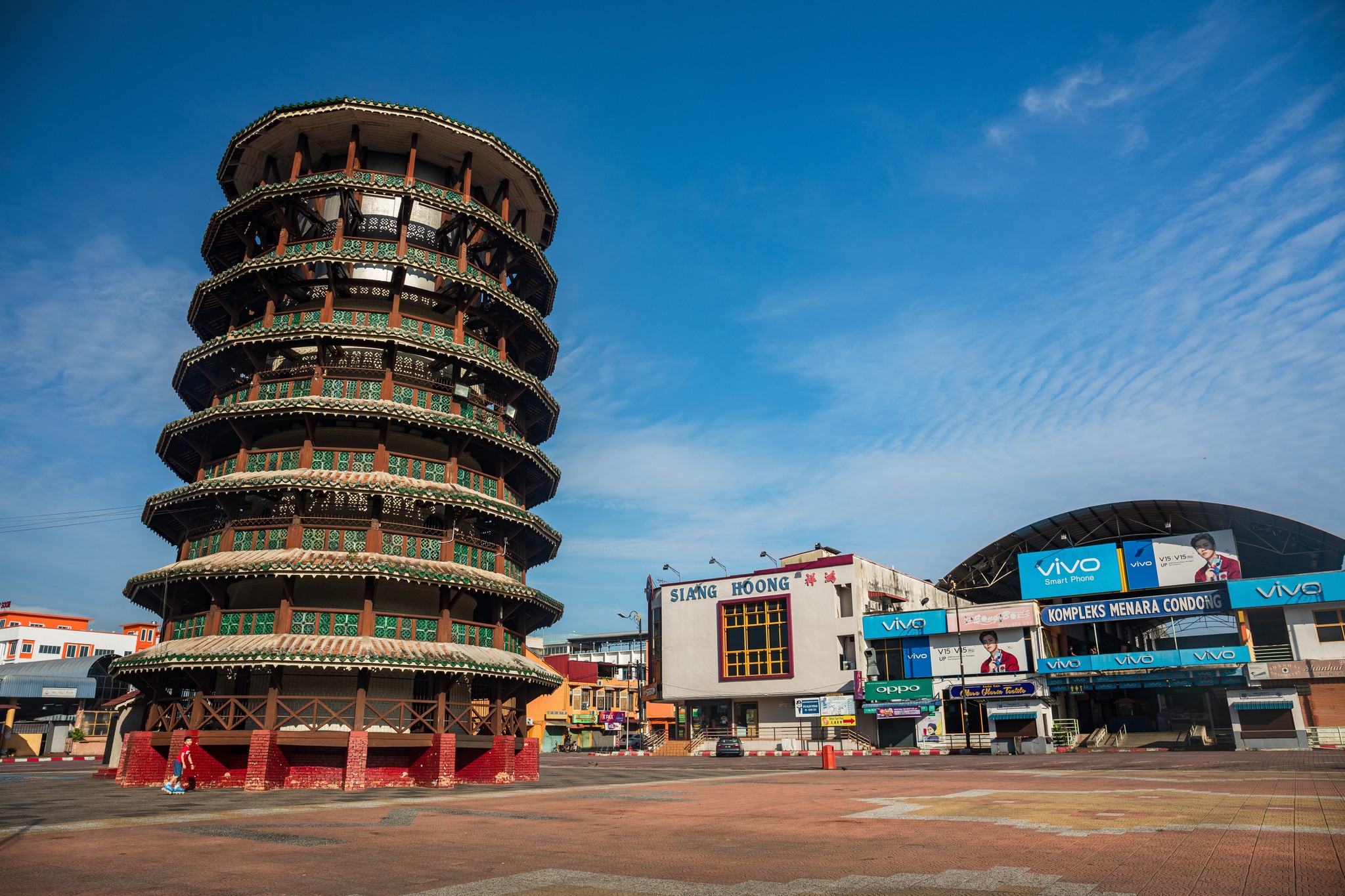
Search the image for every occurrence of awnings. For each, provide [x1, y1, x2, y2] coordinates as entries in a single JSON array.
[[861, 699, 942, 708], [1234, 701, 1294, 710], [569, 723, 584, 728], [625, 719, 647, 723], [546, 722, 574, 726], [989, 711, 1037, 720]]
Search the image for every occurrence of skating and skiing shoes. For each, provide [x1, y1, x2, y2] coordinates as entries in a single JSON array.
[[161, 781, 173, 795], [173, 784, 185, 794]]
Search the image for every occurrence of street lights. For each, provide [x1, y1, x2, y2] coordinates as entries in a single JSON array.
[[617, 611, 645, 749], [937, 577, 975, 755]]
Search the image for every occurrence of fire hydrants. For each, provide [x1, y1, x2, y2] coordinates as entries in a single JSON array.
[[628, 746, 632, 751]]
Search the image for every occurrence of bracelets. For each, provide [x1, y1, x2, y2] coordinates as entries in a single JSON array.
[[190, 763, 193, 765]]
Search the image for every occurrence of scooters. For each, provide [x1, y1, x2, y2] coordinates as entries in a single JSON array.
[[553, 740, 581, 753]]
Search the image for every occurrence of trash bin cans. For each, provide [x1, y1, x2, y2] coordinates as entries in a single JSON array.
[[822, 744, 836, 770]]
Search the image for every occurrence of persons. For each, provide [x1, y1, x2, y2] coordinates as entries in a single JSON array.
[[979, 630, 1019, 674], [712, 718, 717, 738], [161, 736, 194, 794], [922, 723, 939, 741], [1190, 533, 1241, 583], [701, 718, 708, 739], [564, 735, 575, 750]]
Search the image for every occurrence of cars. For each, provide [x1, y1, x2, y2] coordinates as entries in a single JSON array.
[[716, 736, 744, 757], [619, 734, 649, 748]]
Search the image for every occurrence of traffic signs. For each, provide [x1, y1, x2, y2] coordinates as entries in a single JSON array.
[[821, 716, 856, 726]]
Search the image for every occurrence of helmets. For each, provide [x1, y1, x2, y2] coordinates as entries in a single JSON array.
[[565, 735, 568, 737], [568, 735, 571, 737]]
